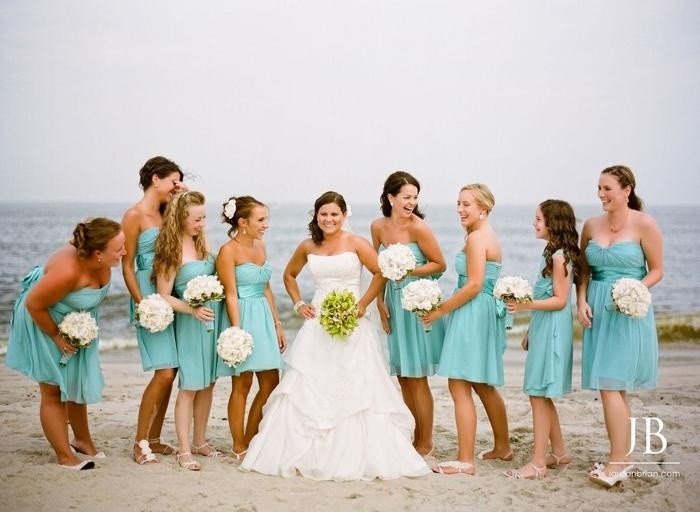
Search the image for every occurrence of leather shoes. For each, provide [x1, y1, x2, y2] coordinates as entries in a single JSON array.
[[69, 445, 106, 458], [57, 460, 95, 470]]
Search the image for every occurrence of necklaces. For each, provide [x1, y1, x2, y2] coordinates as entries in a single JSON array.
[[608, 210, 628, 233]]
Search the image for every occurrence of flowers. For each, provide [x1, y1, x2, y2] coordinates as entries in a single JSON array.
[[215, 324, 256, 372], [489, 272, 535, 306], [375, 241, 418, 282], [318, 288, 371, 342], [399, 276, 447, 337], [133, 290, 175, 336], [604, 275, 653, 321], [53, 306, 99, 368], [182, 272, 226, 333]]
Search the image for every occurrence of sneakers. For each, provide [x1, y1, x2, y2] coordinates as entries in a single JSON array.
[[432, 461, 475, 476]]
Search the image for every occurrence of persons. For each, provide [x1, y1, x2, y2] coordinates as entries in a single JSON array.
[[147, 189, 226, 470], [215, 197, 288, 462], [120, 155, 186, 466], [237, 190, 434, 482], [502, 198, 584, 480], [5, 215, 126, 470], [419, 182, 516, 476], [371, 168, 451, 459], [568, 163, 665, 489]]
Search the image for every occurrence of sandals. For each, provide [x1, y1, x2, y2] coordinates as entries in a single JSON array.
[[177, 441, 225, 470], [548, 452, 570, 469], [502, 462, 549, 480], [589, 462, 634, 490], [132, 438, 178, 465], [477, 449, 515, 461], [228, 448, 248, 464]]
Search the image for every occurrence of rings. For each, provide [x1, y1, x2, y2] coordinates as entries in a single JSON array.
[[205, 311, 210, 316], [64, 345, 68, 351]]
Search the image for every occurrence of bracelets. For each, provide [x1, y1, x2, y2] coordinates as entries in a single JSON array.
[[274, 320, 282, 328], [294, 300, 306, 317]]
